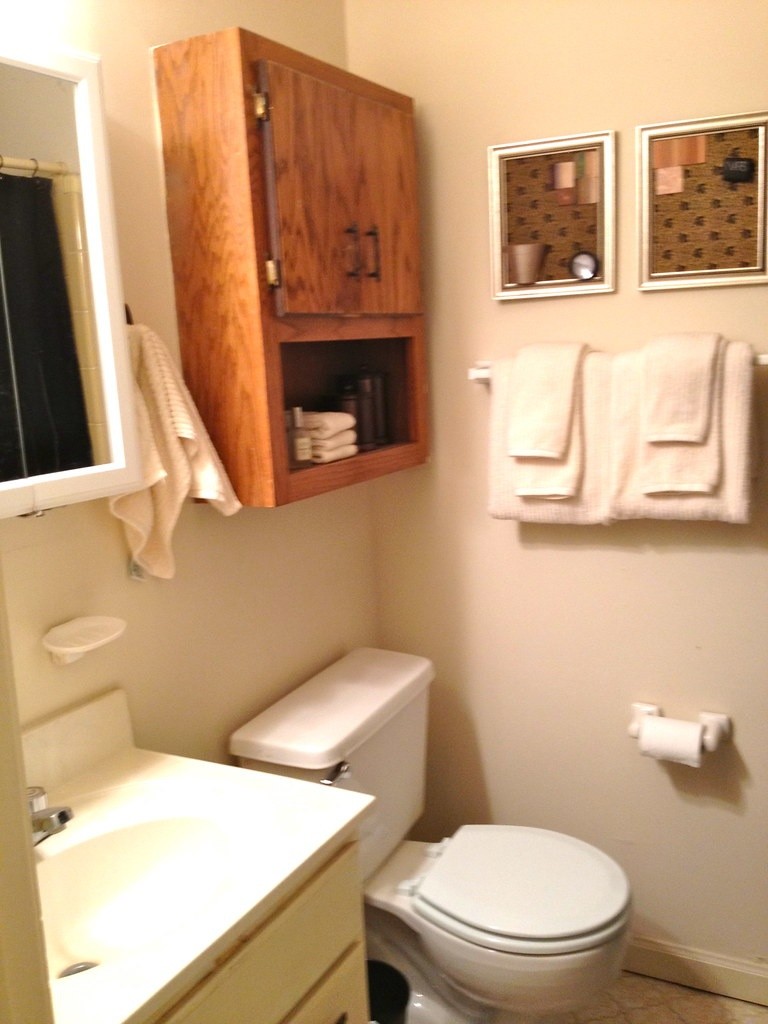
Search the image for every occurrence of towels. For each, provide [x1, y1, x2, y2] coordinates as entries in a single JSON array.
[[109, 322, 242, 579], [489, 329, 610, 534], [607, 329, 754, 528], [286, 412, 358, 464]]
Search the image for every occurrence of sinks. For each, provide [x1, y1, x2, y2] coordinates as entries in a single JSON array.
[[37, 814, 241, 986]]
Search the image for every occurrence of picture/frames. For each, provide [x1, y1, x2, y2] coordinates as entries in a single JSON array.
[[487, 131, 616, 301], [635, 110, 768, 291]]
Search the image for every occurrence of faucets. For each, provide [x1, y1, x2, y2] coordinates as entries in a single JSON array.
[[28, 785, 73, 845]]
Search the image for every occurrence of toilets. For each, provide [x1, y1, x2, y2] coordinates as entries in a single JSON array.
[[232, 650, 634, 1023]]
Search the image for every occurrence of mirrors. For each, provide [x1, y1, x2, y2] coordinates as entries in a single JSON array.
[[0, 58, 112, 485]]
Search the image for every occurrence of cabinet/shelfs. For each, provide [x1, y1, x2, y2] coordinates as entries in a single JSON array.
[[153, 27, 430, 508], [140, 832, 372, 1024]]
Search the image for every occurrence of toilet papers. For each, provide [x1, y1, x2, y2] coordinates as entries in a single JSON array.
[[640, 717, 707, 768]]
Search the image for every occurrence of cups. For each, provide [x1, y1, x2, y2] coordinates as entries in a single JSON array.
[[509, 243, 545, 284]]
